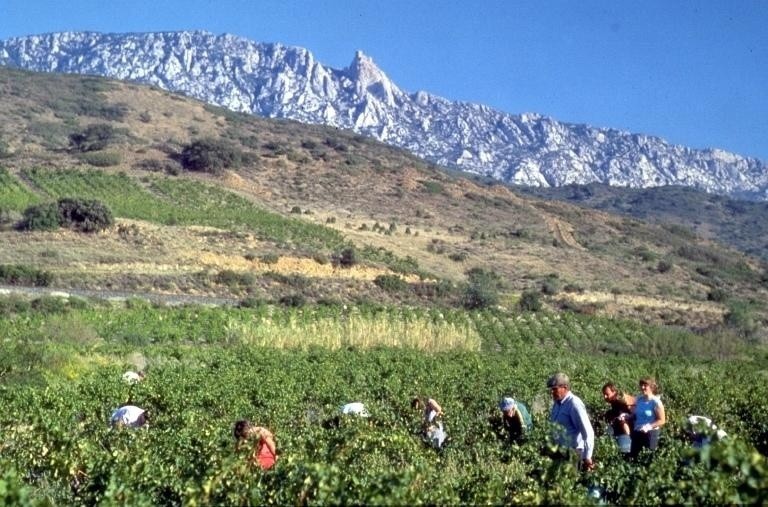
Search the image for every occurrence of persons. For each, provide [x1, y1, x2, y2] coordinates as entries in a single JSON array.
[[112, 404, 151, 426], [683, 415, 727, 447], [499, 395, 531, 435], [411, 395, 447, 445], [602, 380, 636, 439], [617, 375, 668, 456], [233, 420, 279, 467], [546, 373, 598, 480]]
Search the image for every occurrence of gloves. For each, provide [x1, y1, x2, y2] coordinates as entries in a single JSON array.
[[640, 423, 653, 433]]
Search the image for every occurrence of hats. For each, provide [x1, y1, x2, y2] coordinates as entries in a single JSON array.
[[500, 398, 515, 411], [546, 372, 570, 388]]
